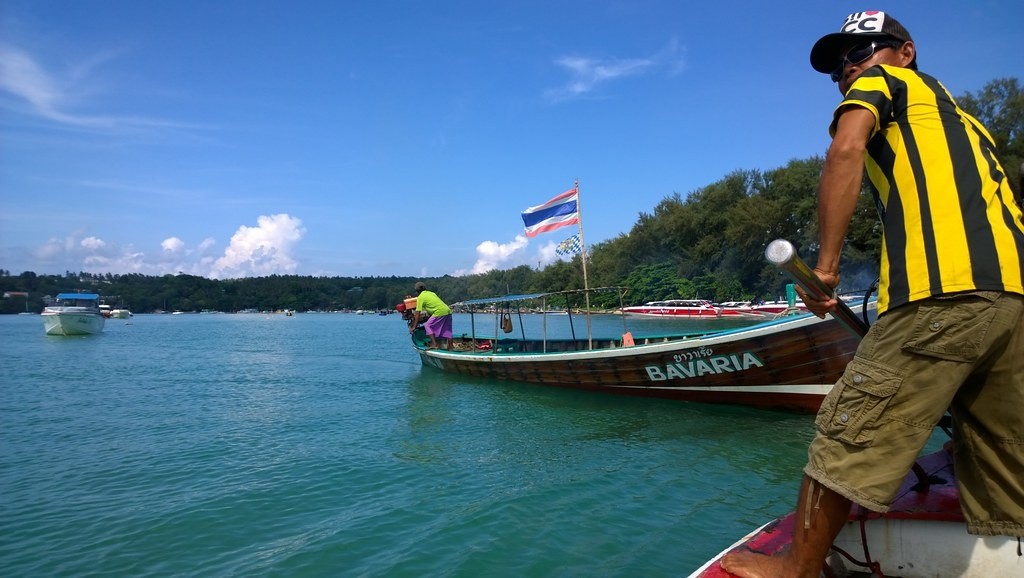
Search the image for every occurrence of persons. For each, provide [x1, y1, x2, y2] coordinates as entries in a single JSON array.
[[719, 10, 1024, 578], [408, 282, 454, 351]]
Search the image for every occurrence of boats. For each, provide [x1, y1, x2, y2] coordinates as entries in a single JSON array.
[[38, 294, 106, 337], [99, 305, 110, 317], [616, 297, 872, 320], [154, 308, 397, 317], [398, 290, 883, 414], [113, 310, 130, 319], [546, 309, 566, 316], [685, 442, 1024, 578]]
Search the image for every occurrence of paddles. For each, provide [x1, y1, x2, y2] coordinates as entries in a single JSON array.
[[766, 237, 956, 417]]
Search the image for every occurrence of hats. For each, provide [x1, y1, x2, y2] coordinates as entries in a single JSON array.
[[811, 11, 913, 74], [415, 282, 425, 289]]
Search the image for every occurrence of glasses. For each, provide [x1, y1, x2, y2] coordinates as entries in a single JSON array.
[[829, 40, 897, 83]]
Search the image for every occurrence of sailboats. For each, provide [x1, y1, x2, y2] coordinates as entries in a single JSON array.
[[20, 301, 35, 315]]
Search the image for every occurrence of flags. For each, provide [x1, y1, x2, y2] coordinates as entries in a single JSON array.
[[554, 234, 582, 257], [520, 187, 578, 238]]
